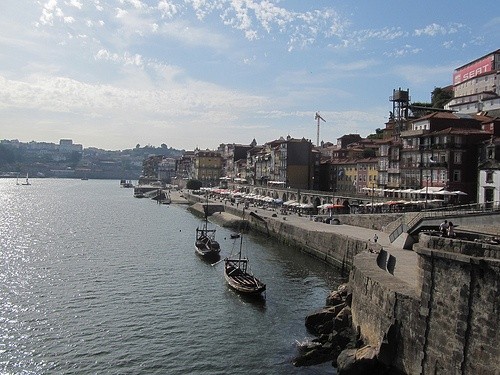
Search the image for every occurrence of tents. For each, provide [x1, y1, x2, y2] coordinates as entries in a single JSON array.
[[212, 188, 344, 210]]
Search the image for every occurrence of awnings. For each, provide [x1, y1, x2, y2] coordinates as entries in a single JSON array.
[[361, 186, 467, 196]]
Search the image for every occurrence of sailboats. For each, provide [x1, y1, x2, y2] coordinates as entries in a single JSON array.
[[16, 173, 32, 185], [193, 190, 221, 257], [224, 207, 266, 297]]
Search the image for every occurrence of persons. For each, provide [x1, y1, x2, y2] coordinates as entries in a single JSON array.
[[373, 233, 378, 242], [439, 220, 455, 239]]
